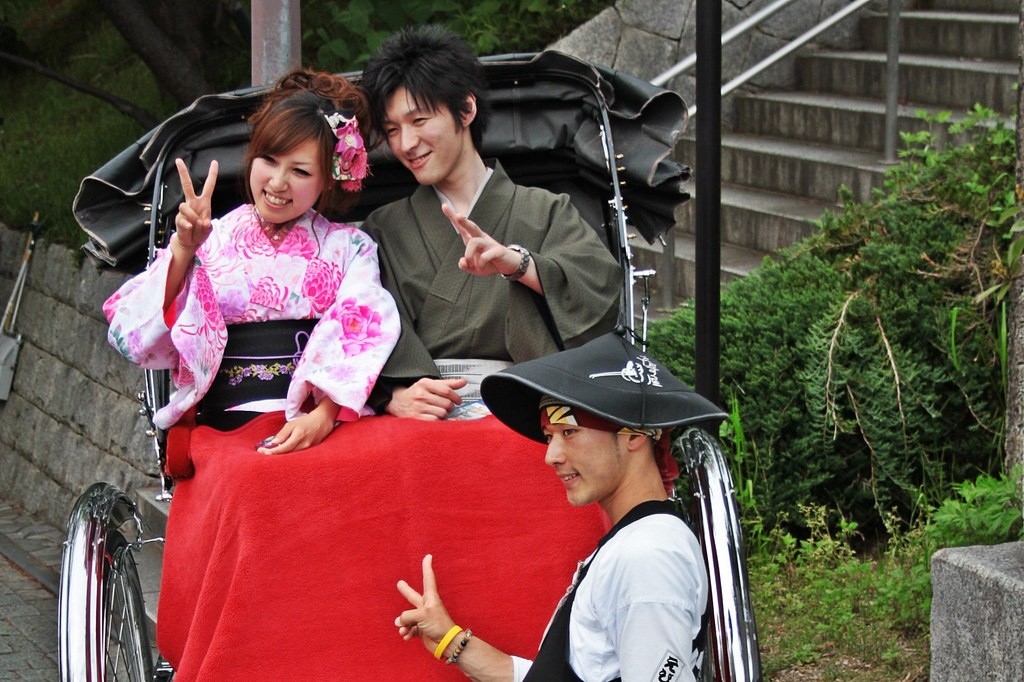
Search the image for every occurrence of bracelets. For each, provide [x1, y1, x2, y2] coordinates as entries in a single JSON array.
[[433, 624, 471, 664]]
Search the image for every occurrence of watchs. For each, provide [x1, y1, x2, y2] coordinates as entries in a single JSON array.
[[501, 242, 532, 280]]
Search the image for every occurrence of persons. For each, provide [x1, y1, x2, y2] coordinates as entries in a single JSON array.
[[394, 331, 735, 682], [361, 24, 625, 425], [100, 67, 400, 454]]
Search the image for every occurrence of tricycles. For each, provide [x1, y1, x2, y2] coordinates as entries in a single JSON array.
[[54, 42, 759, 682]]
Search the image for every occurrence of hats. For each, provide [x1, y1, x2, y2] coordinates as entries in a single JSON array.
[[480, 326, 729, 444]]
